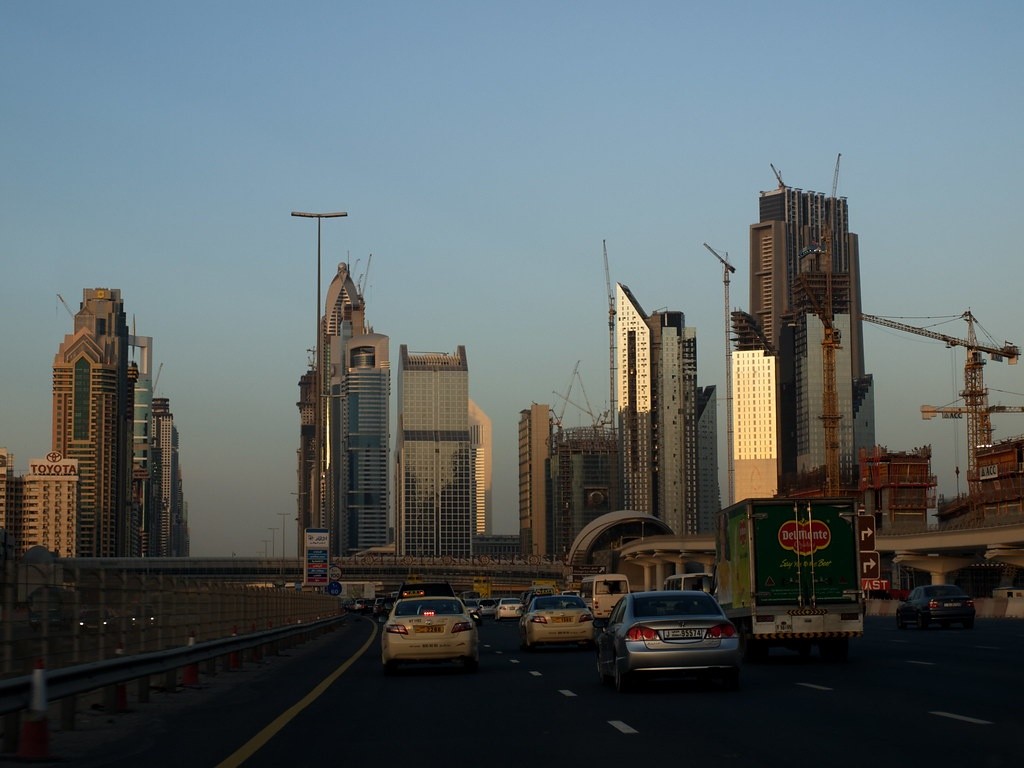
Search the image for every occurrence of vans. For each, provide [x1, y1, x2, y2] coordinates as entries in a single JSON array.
[[663, 572, 715, 597], [579, 573, 631, 620], [559, 590, 581, 609]]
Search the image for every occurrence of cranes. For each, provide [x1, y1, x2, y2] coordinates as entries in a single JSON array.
[[797, 151, 842, 496], [602, 238, 620, 513], [703, 242, 736, 506], [853, 304, 1024, 528]]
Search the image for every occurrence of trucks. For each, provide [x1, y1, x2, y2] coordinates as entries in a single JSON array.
[[701, 496, 867, 653]]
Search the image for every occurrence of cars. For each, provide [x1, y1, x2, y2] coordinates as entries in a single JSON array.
[[349, 581, 483, 624], [354, 594, 479, 676], [494, 597, 527, 622], [895, 584, 976, 630], [518, 593, 597, 651], [475, 598, 498, 618], [592, 590, 743, 687]]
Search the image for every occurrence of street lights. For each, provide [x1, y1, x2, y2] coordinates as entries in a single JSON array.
[[277, 512, 291, 587], [269, 527, 278, 559], [291, 491, 308, 584], [291, 210, 349, 582], [262, 539, 272, 588]]
